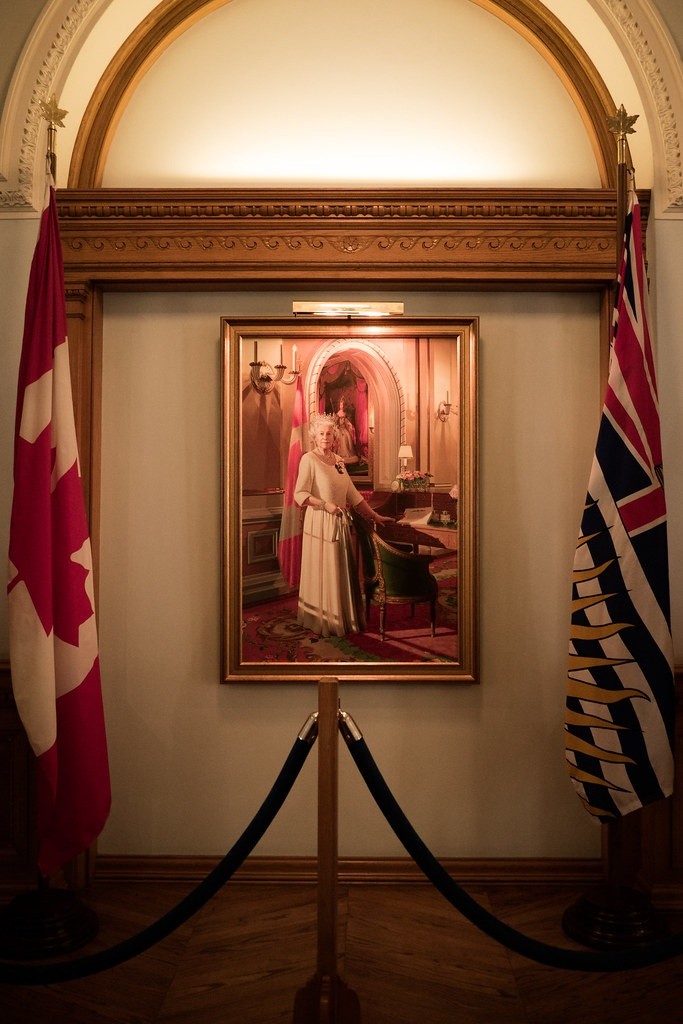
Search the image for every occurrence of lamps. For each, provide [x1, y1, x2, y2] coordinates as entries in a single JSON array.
[[438, 390, 457, 422], [398, 444, 414, 472], [249, 338, 300, 394]]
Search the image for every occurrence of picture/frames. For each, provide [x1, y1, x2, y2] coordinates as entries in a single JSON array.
[[219, 316, 479, 685]]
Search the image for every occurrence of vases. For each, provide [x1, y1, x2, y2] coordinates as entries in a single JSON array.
[[402, 477, 430, 490]]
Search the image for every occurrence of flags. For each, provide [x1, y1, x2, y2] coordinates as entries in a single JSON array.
[[565, 163, 674, 827], [5, 159, 111, 880]]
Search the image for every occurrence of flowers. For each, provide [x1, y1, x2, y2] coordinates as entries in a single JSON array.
[[448, 483, 459, 503], [395, 471, 434, 481]]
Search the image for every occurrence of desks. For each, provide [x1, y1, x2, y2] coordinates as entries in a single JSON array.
[[366, 485, 457, 550]]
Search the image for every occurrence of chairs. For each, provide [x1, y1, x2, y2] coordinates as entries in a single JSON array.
[[357, 516, 447, 642]]
